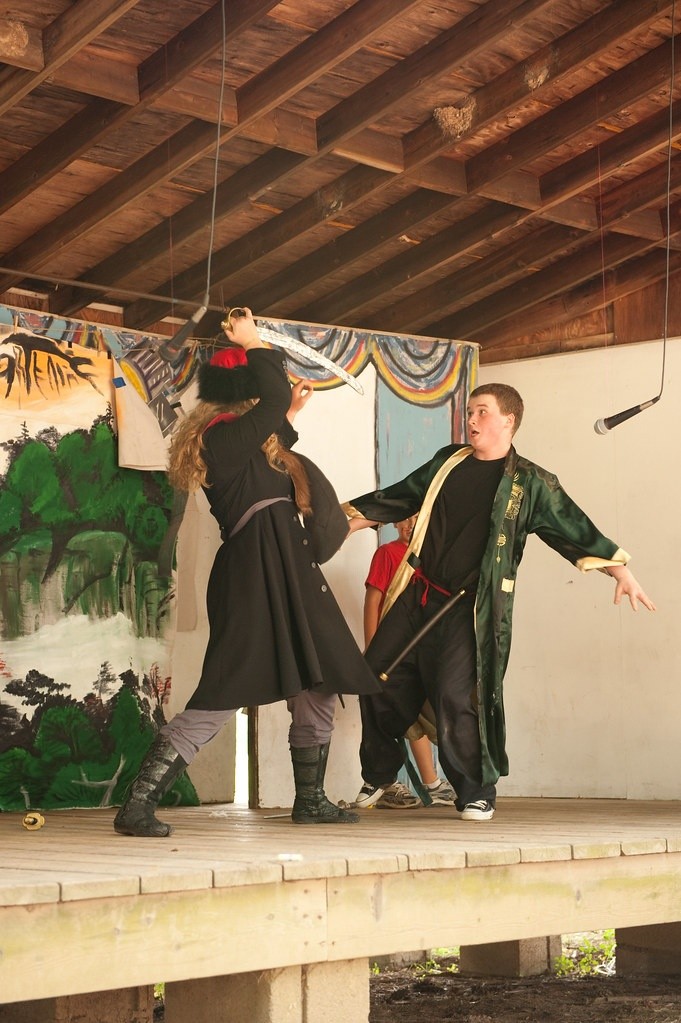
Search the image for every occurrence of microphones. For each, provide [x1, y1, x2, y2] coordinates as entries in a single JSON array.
[[158, 306, 208, 362], [594, 395, 660, 435]]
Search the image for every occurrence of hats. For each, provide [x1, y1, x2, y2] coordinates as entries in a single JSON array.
[[195, 347, 259, 403]]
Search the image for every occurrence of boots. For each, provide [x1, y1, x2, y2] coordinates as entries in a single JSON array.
[[114, 733, 189, 837], [289, 741, 360, 824]]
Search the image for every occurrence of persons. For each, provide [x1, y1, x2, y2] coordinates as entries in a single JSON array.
[[348, 383, 656, 820], [114, 306, 381, 838], [364, 512, 457, 808]]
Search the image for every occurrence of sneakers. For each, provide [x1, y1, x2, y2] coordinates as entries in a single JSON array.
[[425, 781, 458, 806], [461, 800, 495, 820], [375, 780, 422, 809], [355, 779, 398, 808]]
